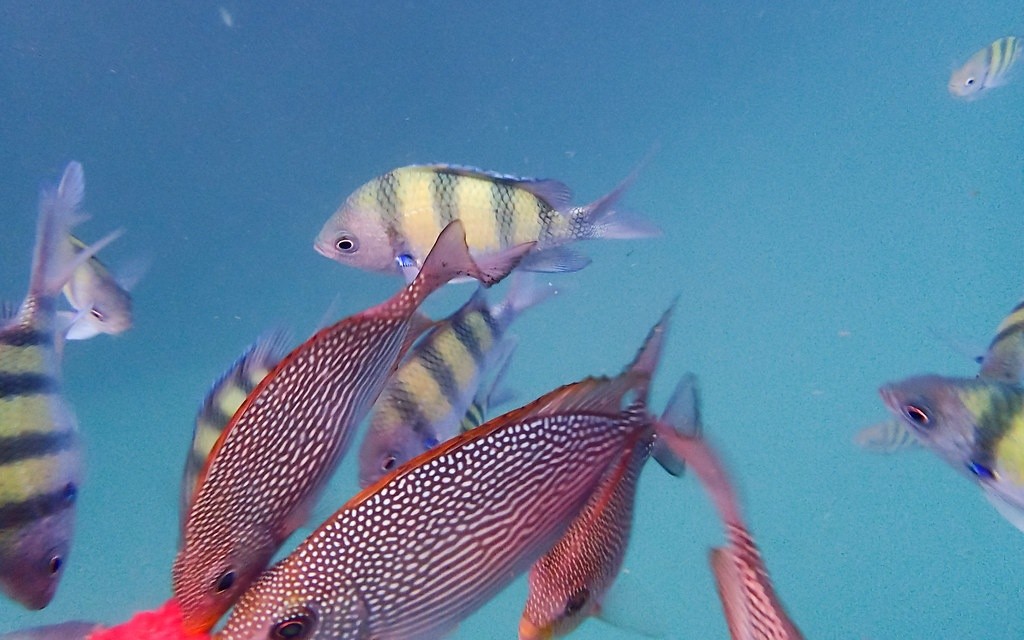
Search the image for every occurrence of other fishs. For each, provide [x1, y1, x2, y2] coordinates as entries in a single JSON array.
[[946, 35, 1024, 97], [311, 161, 662, 286], [0, 162, 1023, 640]]
